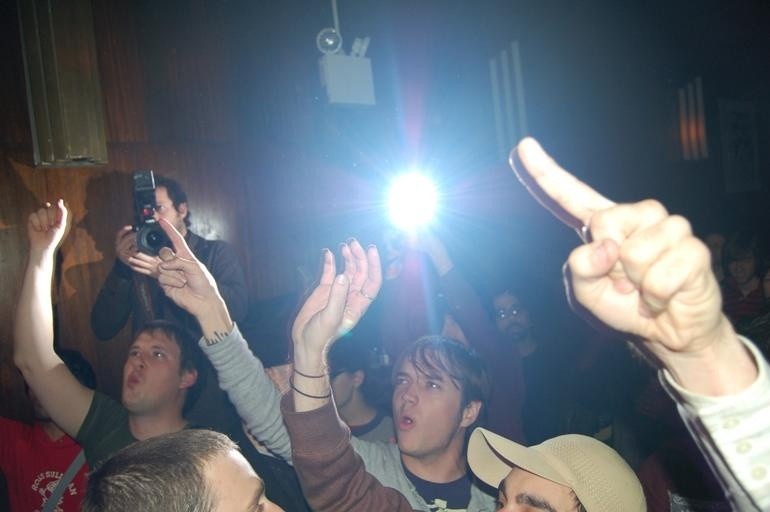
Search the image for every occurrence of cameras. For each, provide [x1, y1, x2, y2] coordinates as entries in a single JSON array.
[[132, 170, 170, 257]]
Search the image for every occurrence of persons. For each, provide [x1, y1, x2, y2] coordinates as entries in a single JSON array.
[[0, 136, 769, 511]]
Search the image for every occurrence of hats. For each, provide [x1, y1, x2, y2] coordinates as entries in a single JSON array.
[[466, 427, 648, 512]]
